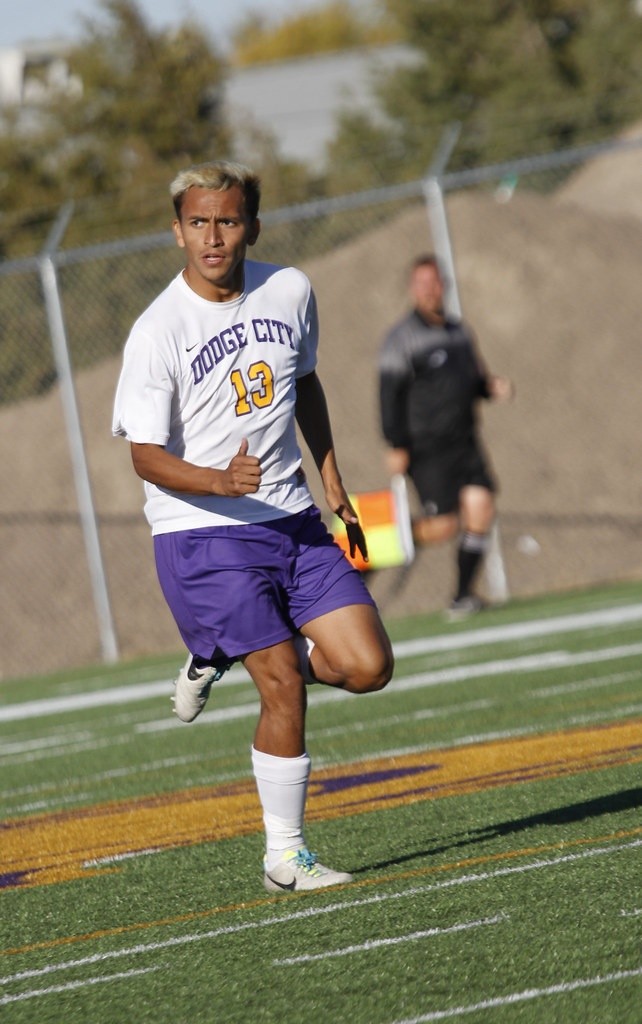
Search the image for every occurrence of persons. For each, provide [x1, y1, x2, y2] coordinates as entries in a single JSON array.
[[110, 164, 393, 894], [372, 255, 511, 613]]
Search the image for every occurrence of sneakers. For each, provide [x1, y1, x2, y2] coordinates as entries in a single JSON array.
[[170, 652, 234, 723], [262, 845, 353, 895]]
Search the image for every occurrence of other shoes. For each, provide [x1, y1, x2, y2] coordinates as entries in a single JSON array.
[[445, 595, 481, 623]]
